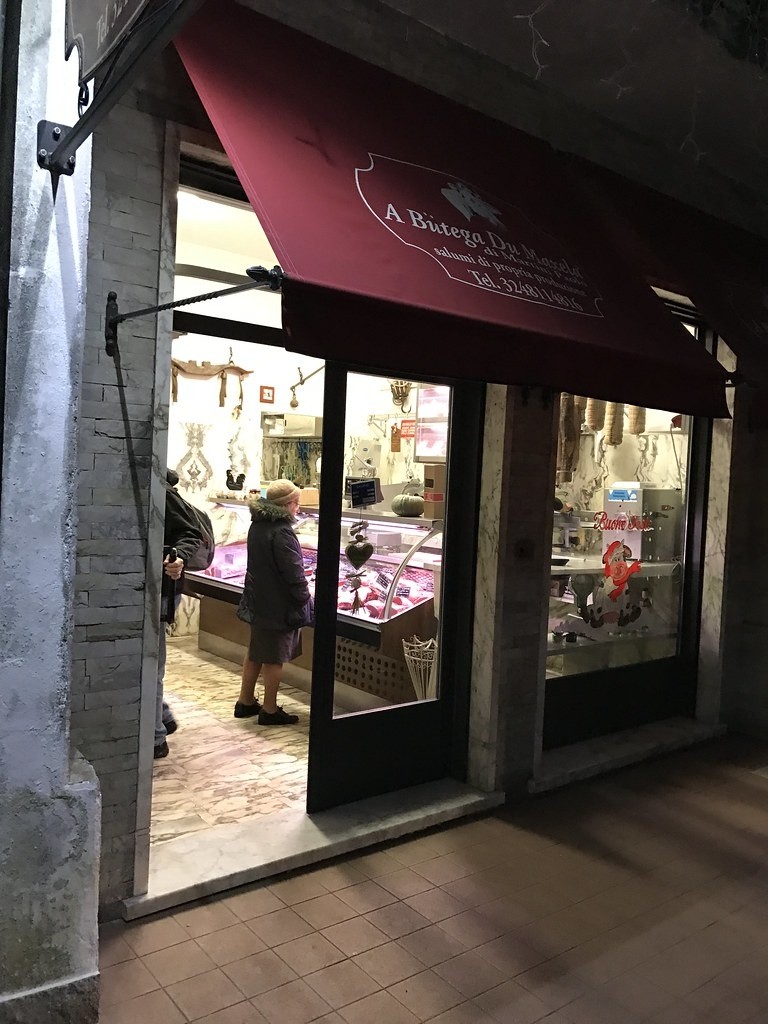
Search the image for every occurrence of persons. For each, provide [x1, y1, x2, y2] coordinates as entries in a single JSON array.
[[553, 497, 573, 514], [154, 468, 203, 759], [234, 479, 312, 726]]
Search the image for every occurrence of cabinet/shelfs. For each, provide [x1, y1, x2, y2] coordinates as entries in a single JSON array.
[[547, 556, 680, 657]]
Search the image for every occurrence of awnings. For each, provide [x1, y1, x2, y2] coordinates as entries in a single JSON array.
[[172, 1, 768, 434]]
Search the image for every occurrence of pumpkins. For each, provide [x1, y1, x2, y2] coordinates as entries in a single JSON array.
[[391, 492, 425, 517]]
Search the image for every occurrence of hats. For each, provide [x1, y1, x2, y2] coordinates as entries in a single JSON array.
[[267, 478, 301, 505]]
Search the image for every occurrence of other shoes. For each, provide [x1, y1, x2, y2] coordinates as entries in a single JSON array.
[[234, 697, 263, 718], [258, 704, 298, 725], [153, 739, 169, 759], [164, 721, 177, 735]]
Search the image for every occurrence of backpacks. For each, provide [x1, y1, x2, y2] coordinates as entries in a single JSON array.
[[166, 480, 215, 571]]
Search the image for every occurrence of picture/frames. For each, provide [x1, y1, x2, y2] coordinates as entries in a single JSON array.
[[260, 385, 274, 403]]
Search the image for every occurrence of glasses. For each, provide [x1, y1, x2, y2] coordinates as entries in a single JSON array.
[[292, 499, 301, 507]]
